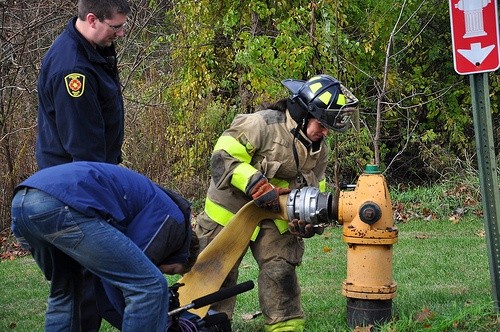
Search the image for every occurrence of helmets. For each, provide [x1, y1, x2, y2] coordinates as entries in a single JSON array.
[[281, 74, 359, 132]]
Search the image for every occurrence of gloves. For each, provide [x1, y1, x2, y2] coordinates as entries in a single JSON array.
[[288, 218, 324, 238], [246, 172, 291, 214]]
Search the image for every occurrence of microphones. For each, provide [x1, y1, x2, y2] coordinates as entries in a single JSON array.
[[168, 280, 255, 317]]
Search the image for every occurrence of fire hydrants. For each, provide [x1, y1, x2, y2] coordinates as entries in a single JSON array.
[[327, 159, 401, 331]]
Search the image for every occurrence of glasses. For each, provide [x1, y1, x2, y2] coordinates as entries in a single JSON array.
[[90, 12, 127, 32]]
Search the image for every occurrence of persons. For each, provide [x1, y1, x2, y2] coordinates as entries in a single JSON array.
[[33, 0, 131, 174], [10, 160, 201, 332], [173, 73, 360, 331]]
